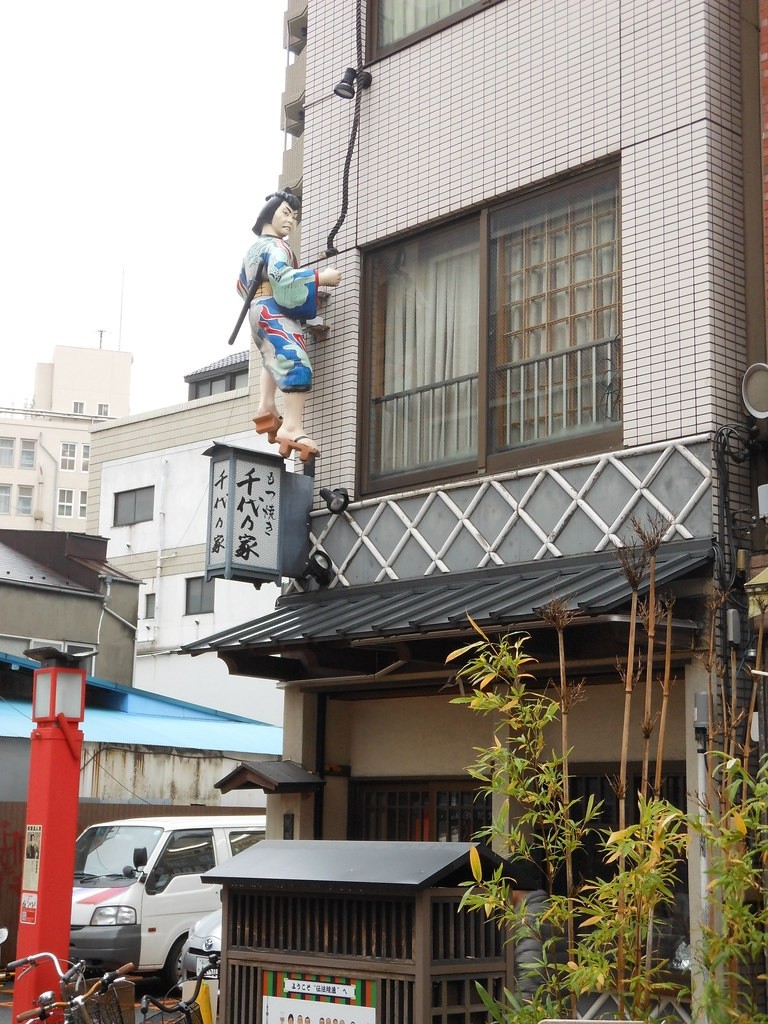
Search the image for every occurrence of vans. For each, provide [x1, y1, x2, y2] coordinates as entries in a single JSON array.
[[68, 815, 266, 997]]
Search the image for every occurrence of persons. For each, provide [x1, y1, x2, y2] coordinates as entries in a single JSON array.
[[236, 187, 341, 461], [504, 858, 569, 1014]]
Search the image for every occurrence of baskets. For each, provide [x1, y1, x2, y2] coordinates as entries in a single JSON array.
[[139, 1002, 203, 1024], [61, 977, 135, 1024], [63, 987, 124, 1024]]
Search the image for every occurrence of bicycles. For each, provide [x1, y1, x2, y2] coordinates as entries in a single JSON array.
[[15, 961, 136, 1024], [6, 951, 87, 1024], [140, 953, 221, 1024]]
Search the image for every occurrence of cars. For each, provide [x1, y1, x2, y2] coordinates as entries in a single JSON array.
[[181, 907, 296, 982]]
